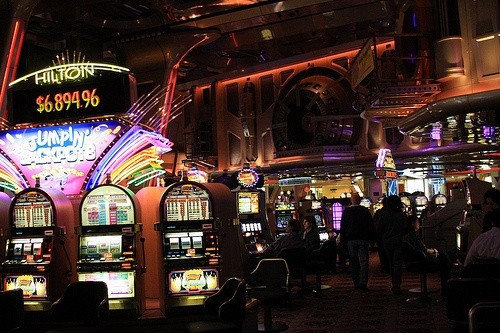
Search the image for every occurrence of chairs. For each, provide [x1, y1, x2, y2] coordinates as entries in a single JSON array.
[[0, 288, 24, 333], [400, 231, 500, 333], [51, 281, 109, 333], [185, 239, 338, 333]]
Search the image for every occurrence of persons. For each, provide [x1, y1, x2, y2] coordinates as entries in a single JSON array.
[[263, 215, 321, 293], [335, 188, 500, 324]]
